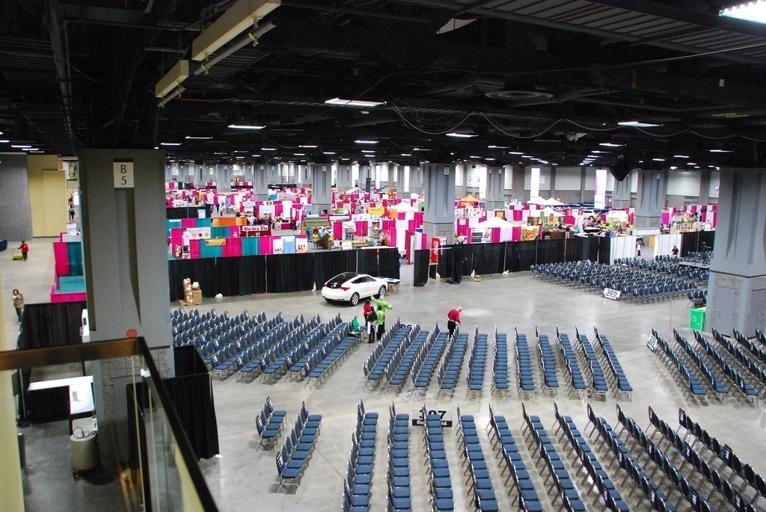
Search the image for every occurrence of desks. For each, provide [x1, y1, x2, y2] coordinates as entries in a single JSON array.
[[67, 430, 99, 481]]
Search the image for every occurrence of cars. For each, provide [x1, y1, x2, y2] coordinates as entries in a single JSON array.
[[321, 271, 388, 307]]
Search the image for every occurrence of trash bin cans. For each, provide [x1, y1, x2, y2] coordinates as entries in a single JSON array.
[[689, 308, 705, 332]]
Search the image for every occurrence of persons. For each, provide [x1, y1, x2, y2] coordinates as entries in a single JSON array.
[[18, 241, 28, 261], [671, 246, 679, 255], [12, 289, 25, 322], [303, 222, 329, 248], [248, 215, 255, 226], [636, 239, 642, 256], [447, 307, 462, 340], [457, 233, 465, 245], [219, 206, 223, 216], [216, 202, 220, 213], [364, 294, 393, 343]]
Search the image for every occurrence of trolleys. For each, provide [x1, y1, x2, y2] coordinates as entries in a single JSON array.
[[11, 247, 23, 261]]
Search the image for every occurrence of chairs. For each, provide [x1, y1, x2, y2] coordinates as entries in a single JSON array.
[[251, 394, 289, 455], [361, 315, 636, 406], [169, 306, 367, 393], [338, 396, 765, 512], [645, 323, 765, 405], [527, 249, 716, 306], [274, 399, 323, 495]]
